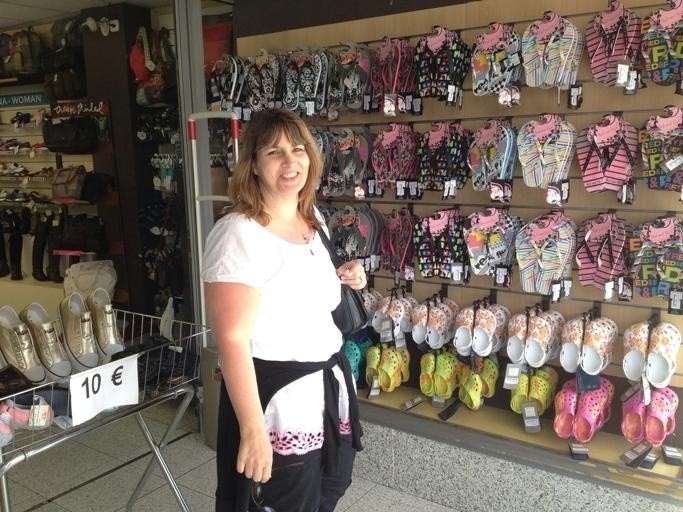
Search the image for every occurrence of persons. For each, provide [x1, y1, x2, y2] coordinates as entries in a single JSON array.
[[202, 108, 368, 512]]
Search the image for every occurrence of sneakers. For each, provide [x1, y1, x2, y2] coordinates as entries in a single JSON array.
[[86, 287, 126, 361], [57, 291, 100, 374], [19, 304, 74, 380], [0, 303, 48, 387], [1, 1, 190, 212]]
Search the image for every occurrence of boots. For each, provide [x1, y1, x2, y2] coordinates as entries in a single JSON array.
[[0, 218, 114, 284]]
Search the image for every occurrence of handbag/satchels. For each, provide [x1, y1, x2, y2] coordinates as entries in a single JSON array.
[[310, 218, 370, 335]]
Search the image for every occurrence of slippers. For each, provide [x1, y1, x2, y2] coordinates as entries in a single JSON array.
[[515, 104, 683, 193], [321, 207, 517, 280], [206, 25, 470, 113], [465, 0, 683, 98], [313, 119, 513, 199], [337, 289, 511, 410], [515, 209, 683, 299], [506, 306, 681, 448]]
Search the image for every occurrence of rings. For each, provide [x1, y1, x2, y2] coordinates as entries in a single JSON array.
[[359, 277, 363, 283]]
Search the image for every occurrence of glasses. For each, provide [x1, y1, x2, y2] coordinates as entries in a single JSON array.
[[250, 460, 306, 512]]
